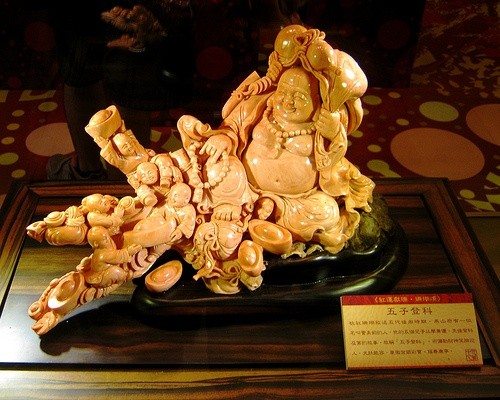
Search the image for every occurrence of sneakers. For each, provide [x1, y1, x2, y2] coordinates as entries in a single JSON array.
[[44, 152, 107, 185]]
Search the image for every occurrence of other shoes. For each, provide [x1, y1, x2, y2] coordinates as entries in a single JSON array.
[[370, 39, 414, 92]]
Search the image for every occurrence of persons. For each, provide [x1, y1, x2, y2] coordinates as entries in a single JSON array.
[[45, 0, 139, 181], [102, 3, 162, 46], [25, 23, 377, 289]]
